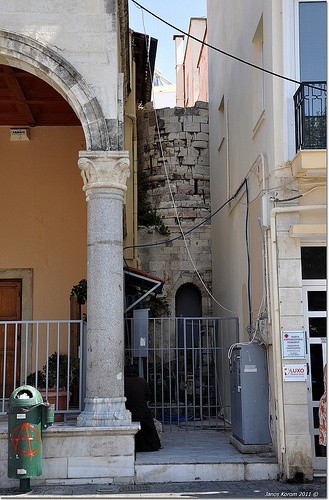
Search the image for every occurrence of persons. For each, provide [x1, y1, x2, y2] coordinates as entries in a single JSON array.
[[122, 361, 162, 453], [316, 363, 327, 448]]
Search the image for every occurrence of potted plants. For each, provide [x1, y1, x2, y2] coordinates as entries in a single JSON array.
[[32, 351, 78, 423]]
